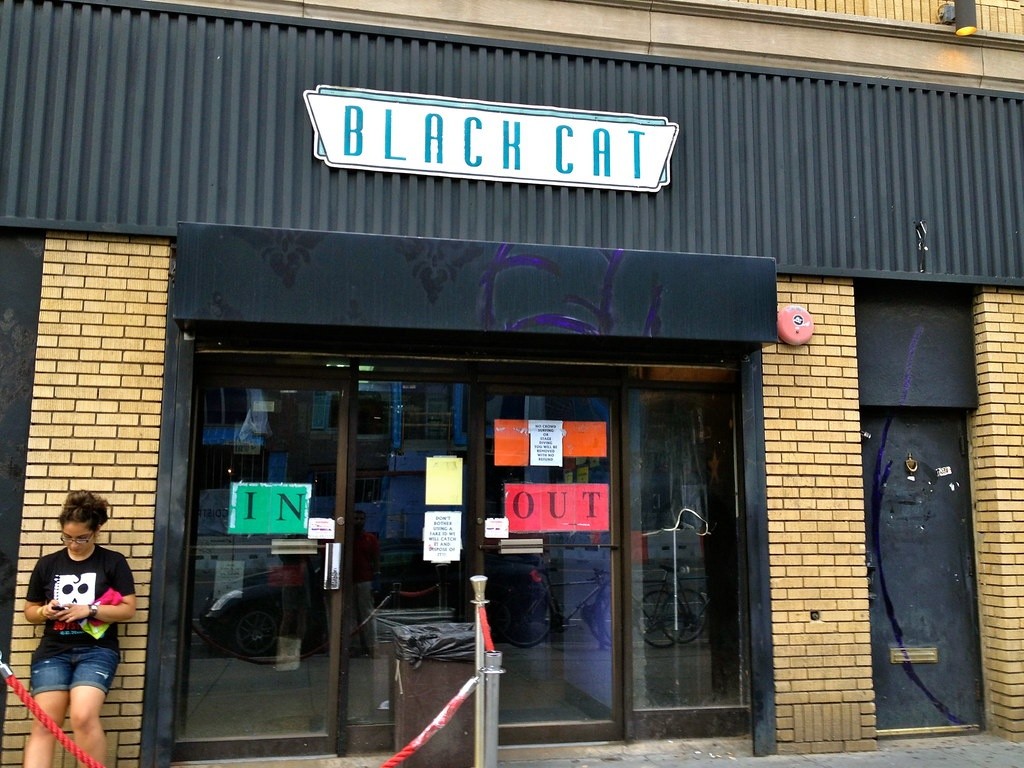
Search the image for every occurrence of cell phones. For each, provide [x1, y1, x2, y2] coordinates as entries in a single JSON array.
[[52, 606, 65, 611]]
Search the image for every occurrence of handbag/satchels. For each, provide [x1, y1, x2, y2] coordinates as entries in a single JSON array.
[[267, 564, 304, 588]]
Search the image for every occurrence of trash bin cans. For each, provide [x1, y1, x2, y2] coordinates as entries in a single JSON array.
[[387, 619, 499, 768]]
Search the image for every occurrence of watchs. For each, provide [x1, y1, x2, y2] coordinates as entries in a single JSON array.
[[88, 604, 98, 616]]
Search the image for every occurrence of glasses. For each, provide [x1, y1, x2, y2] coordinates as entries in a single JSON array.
[[60, 532, 94, 544]]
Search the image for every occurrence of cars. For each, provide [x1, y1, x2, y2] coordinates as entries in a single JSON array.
[[197, 534, 555, 658]]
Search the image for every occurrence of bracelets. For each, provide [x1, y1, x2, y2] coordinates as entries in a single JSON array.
[[37, 606, 48, 620]]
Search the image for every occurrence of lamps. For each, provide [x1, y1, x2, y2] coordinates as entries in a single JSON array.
[[939, 0, 976, 37]]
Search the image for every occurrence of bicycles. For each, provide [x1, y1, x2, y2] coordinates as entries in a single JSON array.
[[499, 556, 709, 648]]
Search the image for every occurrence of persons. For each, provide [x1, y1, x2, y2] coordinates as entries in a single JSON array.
[[350, 508, 385, 659], [26, 488, 138, 768]]
